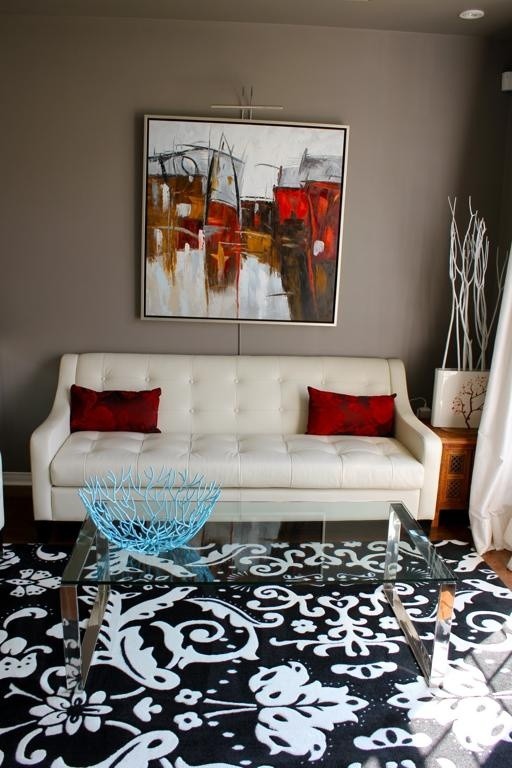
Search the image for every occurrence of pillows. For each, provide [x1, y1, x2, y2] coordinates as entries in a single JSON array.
[[305, 385, 398, 438], [68, 383, 161, 434]]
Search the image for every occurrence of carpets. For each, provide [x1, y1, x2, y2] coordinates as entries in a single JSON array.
[[0, 538, 510, 768]]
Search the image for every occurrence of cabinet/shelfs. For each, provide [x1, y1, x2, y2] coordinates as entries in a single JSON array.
[[420, 418, 478, 530]]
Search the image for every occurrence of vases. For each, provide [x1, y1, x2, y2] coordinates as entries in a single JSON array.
[[432, 366, 491, 430]]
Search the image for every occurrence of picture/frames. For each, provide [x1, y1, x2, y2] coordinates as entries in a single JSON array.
[[141, 112, 351, 326]]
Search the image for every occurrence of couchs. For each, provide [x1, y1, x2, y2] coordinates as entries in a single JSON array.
[[28, 351, 444, 525]]
